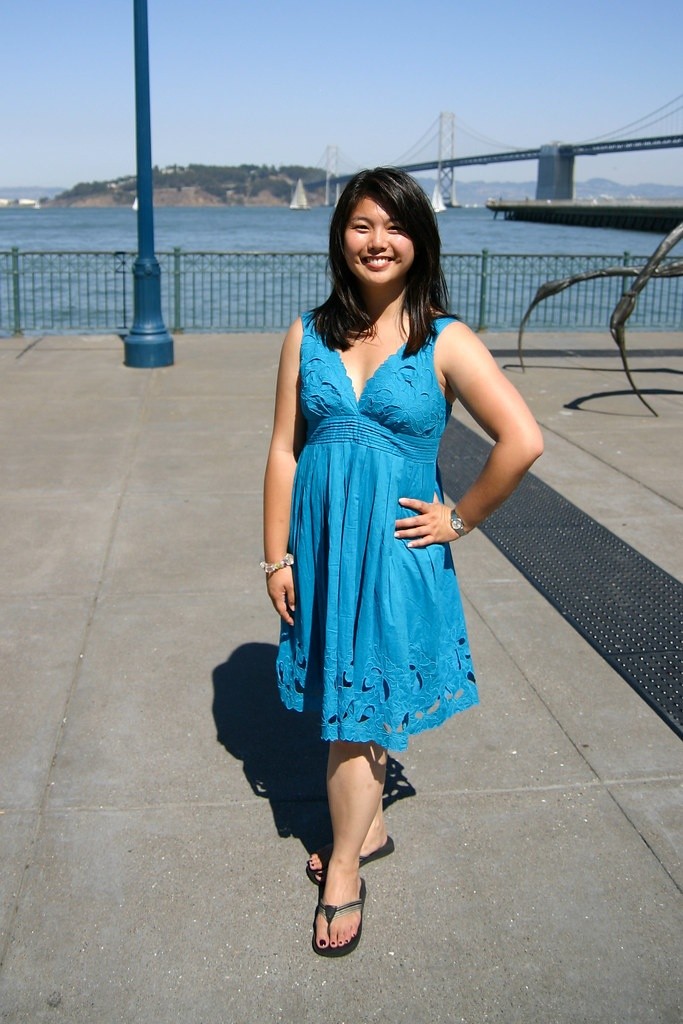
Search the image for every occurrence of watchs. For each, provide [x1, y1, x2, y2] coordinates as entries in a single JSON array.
[[450, 509, 466, 536]]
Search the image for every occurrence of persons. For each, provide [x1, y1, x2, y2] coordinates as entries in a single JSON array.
[[260, 165, 542, 957]]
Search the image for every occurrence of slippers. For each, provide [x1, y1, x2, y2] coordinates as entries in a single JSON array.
[[311, 879, 367, 957], [305, 835, 396, 884]]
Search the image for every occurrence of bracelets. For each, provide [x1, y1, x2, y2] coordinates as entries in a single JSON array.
[[260, 552, 294, 579]]
